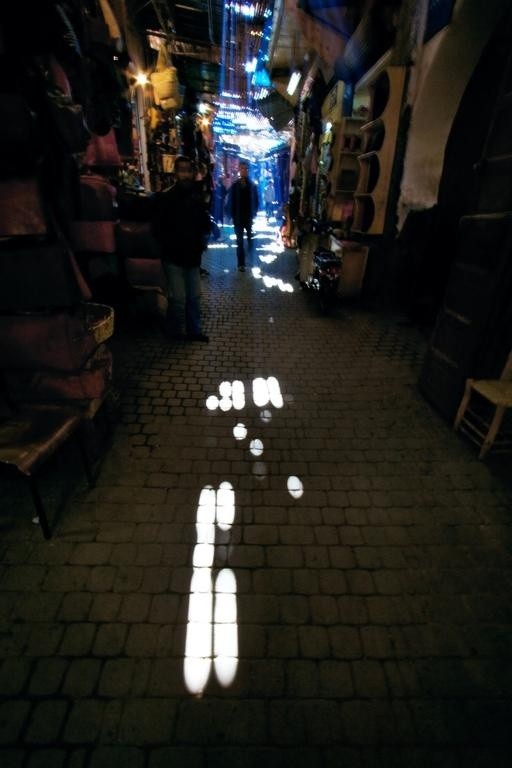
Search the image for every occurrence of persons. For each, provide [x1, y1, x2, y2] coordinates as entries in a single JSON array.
[[247, 175, 258, 236], [231, 162, 259, 272], [201, 162, 275, 226], [159, 157, 213, 343]]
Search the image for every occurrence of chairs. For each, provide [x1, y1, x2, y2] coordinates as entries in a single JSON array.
[[1, 405, 97, 540], [451, 349, 511, 462]]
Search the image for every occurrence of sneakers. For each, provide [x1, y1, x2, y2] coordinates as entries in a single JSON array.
[[171, 334, 209, 343], [239, 266, 246, 272]]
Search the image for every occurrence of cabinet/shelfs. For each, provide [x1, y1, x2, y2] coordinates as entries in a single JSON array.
[[326, 47, 406, 235]]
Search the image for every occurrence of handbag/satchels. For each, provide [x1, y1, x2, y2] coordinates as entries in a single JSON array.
[[0, 300, 115, 398], [150, 66, 185, 111]]
